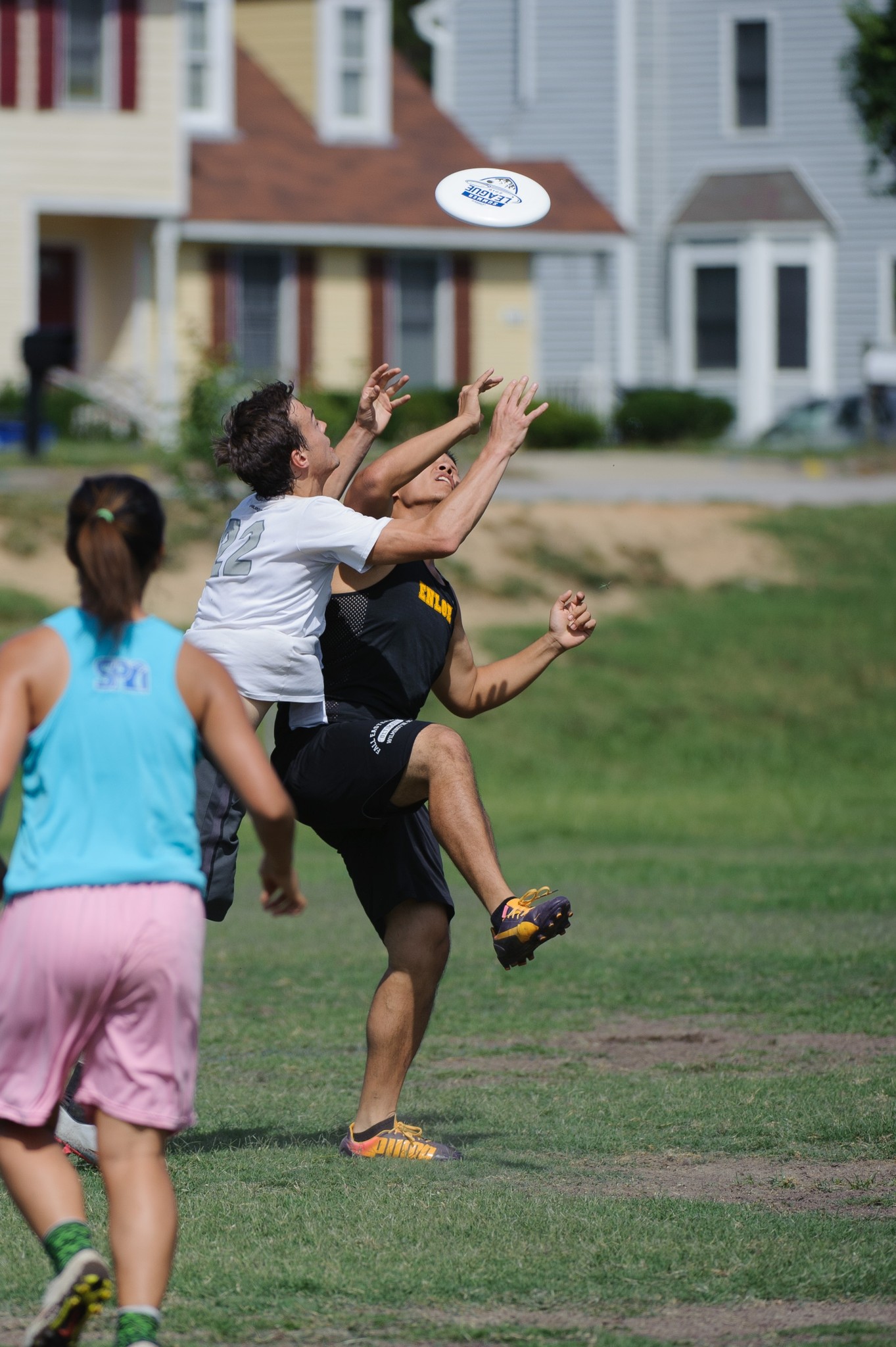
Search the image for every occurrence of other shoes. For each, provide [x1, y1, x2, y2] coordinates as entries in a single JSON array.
[[52, 1060, 101, 1168], [22, 1248, 112, 1346]]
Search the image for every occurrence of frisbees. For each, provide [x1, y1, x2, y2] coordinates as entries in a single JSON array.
[[434, 166, 553, 230]]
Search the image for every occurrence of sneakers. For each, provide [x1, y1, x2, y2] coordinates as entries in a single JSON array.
[[490, 886, 574, 970], [338, 1114, 465, 1165]]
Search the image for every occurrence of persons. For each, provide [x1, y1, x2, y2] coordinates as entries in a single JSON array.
[[858, 340, 896, 435], [0, 468, 308, 1347], [55, 361, 597, 1169], [19, 307, 76, 460]]
